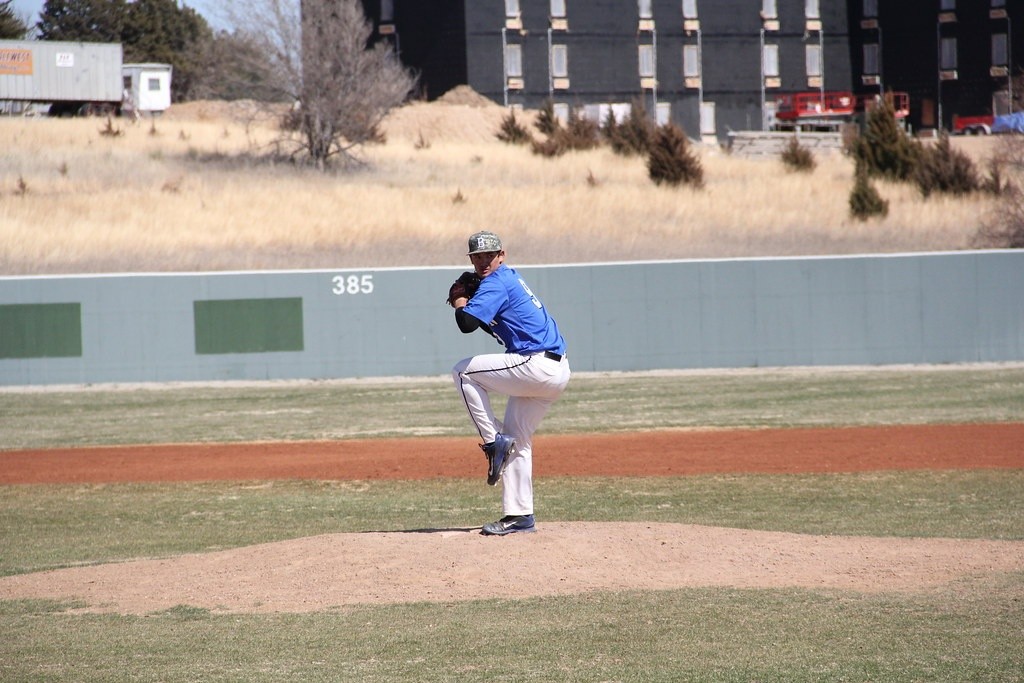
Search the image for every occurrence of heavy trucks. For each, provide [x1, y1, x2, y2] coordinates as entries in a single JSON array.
[[0, 40, 123, 119]]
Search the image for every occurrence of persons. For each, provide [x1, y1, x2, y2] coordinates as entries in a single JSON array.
[[445, 230, 571, 536]]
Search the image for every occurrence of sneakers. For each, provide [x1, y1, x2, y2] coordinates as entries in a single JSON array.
[[478, 431, 516, 486], [481, 515, 536, 534]]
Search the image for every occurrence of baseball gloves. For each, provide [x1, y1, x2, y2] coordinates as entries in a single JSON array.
[[449, 271, 481, 308]]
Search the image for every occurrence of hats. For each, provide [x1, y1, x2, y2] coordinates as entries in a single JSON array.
[[466, 230, 502, 254]]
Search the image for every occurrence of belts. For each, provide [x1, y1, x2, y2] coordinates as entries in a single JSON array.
[[545, 351, 567, 362]]
